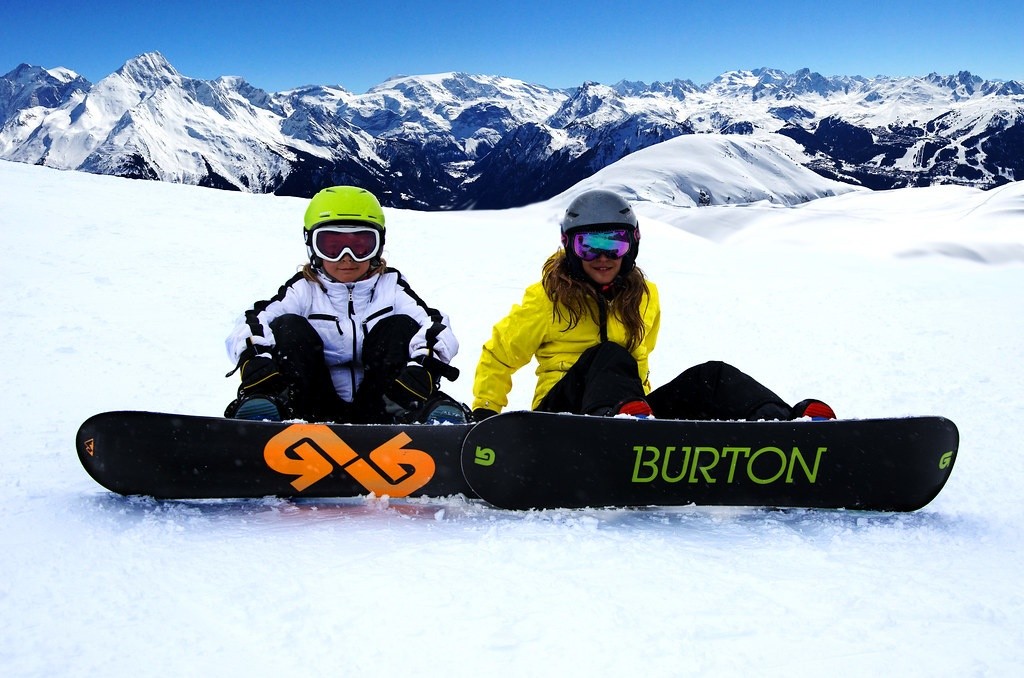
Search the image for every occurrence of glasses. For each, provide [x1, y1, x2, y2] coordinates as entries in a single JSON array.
[[311, 224, 382, 263], [573, 229, 633, 260]]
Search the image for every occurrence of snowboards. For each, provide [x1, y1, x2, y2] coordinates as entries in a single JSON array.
[[74, 410, 476, 501], [462, 410, 960, 511]]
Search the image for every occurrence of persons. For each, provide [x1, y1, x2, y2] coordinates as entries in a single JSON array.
[[224, 185, 470, 425], [472, 189, 837, 421]]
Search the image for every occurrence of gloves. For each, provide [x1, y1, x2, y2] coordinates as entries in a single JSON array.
[[365, 366, 437, 425], [226, 351, 301, 423]]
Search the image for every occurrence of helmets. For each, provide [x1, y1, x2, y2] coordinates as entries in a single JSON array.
[[561, 189, 641, 300], [305, 185, 387, 277]]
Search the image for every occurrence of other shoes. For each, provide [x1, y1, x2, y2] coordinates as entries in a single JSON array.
[[425, 401, 466, 426], [233, 394, 282, 421]]
